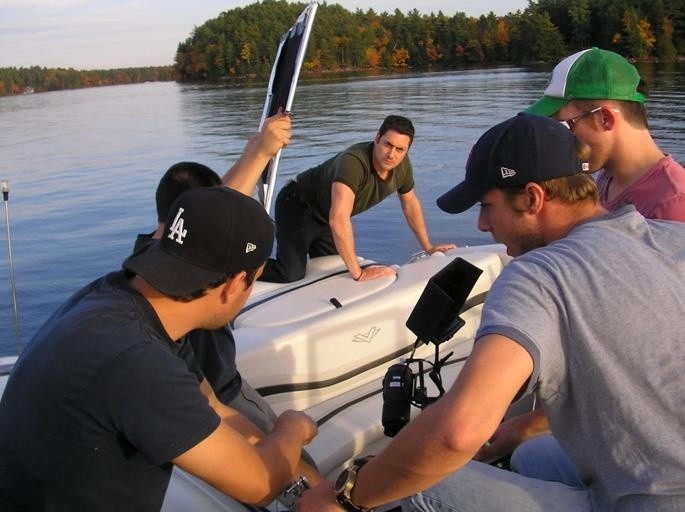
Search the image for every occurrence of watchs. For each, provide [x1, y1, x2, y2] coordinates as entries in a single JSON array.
[[333, 453, 382, 511]]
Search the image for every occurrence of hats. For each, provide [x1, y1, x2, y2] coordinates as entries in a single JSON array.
[[122, 187, 274, 297], [437, 112, 590, 214], [524, 46, 647, 116]]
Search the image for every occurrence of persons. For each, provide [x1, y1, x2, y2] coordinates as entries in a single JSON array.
[[252, 115, 460, 287], [122, 104, 323, 481], [291, 113, 684, 512], [519, 47, 685, 224], [1, 183, 329, 511]]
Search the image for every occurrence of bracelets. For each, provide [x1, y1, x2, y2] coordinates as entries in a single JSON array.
[[352, 267, 364, 281], [279, 474, 312, 505]]
